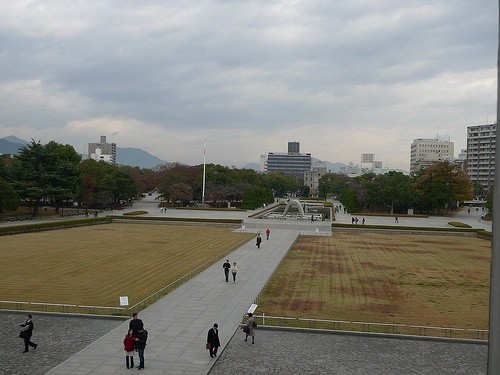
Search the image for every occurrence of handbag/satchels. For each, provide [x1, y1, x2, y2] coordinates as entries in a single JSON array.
[[243, 326, 250, 333], [253, 321, 257, 327], [206, 343, 212, 349], [19, 331, 30, 338]]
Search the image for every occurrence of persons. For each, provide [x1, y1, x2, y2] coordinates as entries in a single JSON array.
[[311, 215, 314, 223], [128, 313, 143, 352], [231, 262, 237, 283], [161, 206, 167, 212], [18, 314, 38, 352], [124, 326, 146, 370], [257, 234, 261, 248], [395, 215, 399, 223], [266, 228, 270, 240], [245, 313, 255, 344], [223, 260, 231, 281], [334, 205, 349, 215], [206, 323, 219, 357], [352, 216, 365, 224], [85, 209, 98, 217]]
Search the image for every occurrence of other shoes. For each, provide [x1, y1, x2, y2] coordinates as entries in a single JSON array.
[[137, 365, 140, 368], [138, 367, 144, 370], [245, 339, 247, 341], [214, 354, 217, 356], [33, 344, 37, 350], [252, 341, 254, 344], [23, 350, 29, 352], [211, 355, 214, 357]]
